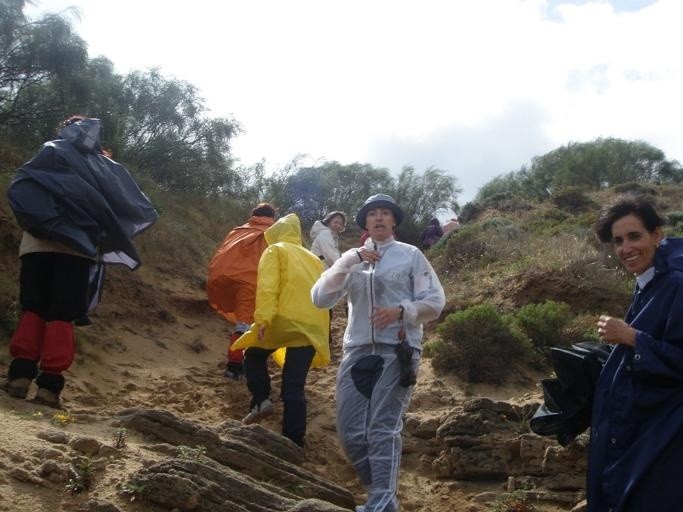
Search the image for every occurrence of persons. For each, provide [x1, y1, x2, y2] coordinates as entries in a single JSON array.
[[309, 193, 447, 511], [306, 211, 348, 270], [207, 202, 282, 381], [3, 113, 161, 407], [583, 196, 683, 511], [236, 211, 333, 447]]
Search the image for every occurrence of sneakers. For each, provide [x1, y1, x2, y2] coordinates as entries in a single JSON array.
[[243, 400, 273, 425]]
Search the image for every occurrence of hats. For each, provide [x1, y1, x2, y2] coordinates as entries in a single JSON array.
[[356, 193, 403, 230]]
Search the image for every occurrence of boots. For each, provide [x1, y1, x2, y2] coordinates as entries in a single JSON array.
[[0, 359, 37, 398], [34, 373, 64, 405]]
